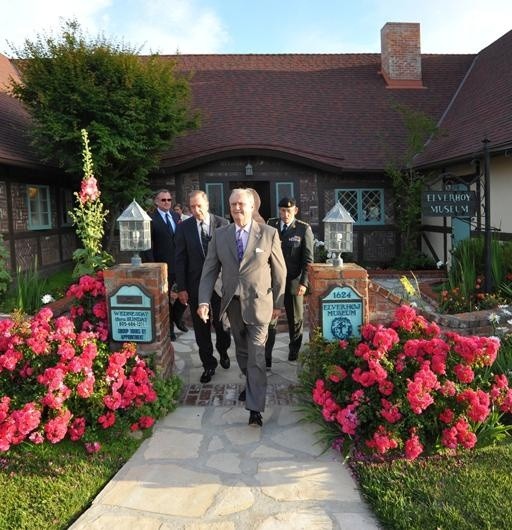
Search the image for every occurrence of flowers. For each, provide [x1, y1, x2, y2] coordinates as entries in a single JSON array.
[[1, 270, 158, 452], [68, 127, 115, 279], [311, 304, 511, 466]]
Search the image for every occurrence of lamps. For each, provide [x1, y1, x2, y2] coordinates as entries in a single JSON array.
[[116, 198, 153, 265], [322, 199, 355, 268]]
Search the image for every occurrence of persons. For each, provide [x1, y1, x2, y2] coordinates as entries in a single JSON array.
[[170, 202, 190, 227], [244, 187, 266, 223], [138, 188, 189, 341], [170, 189, 232, 384], [261, 195, 315, 371], [195, 188, 289, 428]]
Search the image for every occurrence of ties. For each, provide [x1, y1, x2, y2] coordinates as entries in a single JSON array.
[[200, 223, 209, 255], [165, 214, 174, 238], [281, 225, 287, 233], [238, 229, 244, 258]]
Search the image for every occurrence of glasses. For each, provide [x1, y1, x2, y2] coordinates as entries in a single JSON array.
[[161, 198, 171, 202]]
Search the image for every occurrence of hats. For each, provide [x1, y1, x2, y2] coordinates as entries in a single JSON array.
[[279, 198, 295, 207]]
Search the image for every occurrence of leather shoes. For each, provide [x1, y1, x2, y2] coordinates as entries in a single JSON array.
[[249, 414, 263, 428], [240, 390, 245, 401], [170, 322, 176, 341], [216, 345, 230, 369], [288, 351, 298, 361], [171, 314, 188, 332], [200, 368, 215, 383]]
